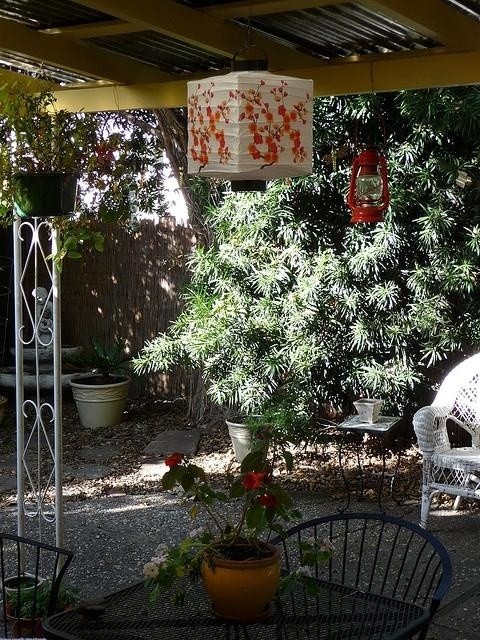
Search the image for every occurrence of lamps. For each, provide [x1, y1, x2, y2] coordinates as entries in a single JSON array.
[[348, 34, 389, 223], [187, 1, 313, 192]]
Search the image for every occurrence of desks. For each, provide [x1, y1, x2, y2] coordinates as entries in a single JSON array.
[[336, 414, 402, 513], [42, 550, 429, 639]]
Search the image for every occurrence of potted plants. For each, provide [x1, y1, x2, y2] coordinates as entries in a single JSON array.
[[62, 334, 134, 429], [4, 578, 75, 639], [226, 411, 290, 468], [0, 73, 102, 217]]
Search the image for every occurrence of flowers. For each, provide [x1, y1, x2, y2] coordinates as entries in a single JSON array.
[[142, 449, 335, 607]]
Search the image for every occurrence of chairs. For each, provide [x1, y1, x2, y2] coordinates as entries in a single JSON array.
[[413, 352, 480, 529], [1, 535, 72, 639], [269, 513, 453, 639]]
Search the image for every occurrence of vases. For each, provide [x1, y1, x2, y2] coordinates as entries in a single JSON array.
[[201, 539, 281, 621], [4, 574, 44, 598], [353, 397, 385, 422]]
